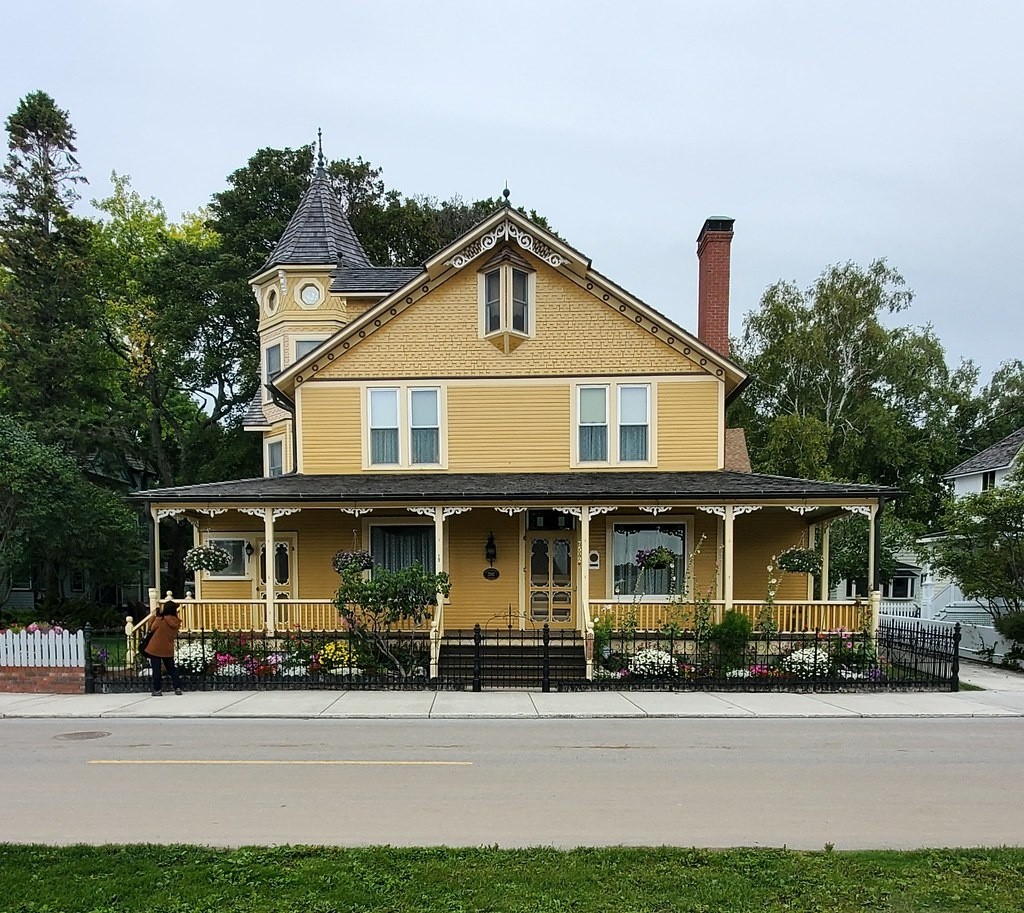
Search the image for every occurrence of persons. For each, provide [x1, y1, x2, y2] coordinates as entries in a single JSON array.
[[145, 602, 183, 696]]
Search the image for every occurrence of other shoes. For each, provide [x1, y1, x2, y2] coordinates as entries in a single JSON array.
[[152, 690, 162, 696], [175, 688, 182, 695]]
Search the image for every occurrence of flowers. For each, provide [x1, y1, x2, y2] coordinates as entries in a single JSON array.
[[775, 545, 824, 577], [332, 548, 375, 574], [634, 546, 677, 570], [89, 644, 110, 664], [182, 539, 233, 574]]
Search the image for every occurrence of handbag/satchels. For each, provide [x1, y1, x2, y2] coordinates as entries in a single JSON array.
[[138, 626, 154, 658]]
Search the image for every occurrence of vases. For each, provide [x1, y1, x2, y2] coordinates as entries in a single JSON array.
[[652, 563, 667, 569], [91, 662, 106, 679]]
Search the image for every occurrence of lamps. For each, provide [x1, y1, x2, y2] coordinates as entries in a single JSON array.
[[245, 541, 254, 563], [485, 531, 497, 567]]
